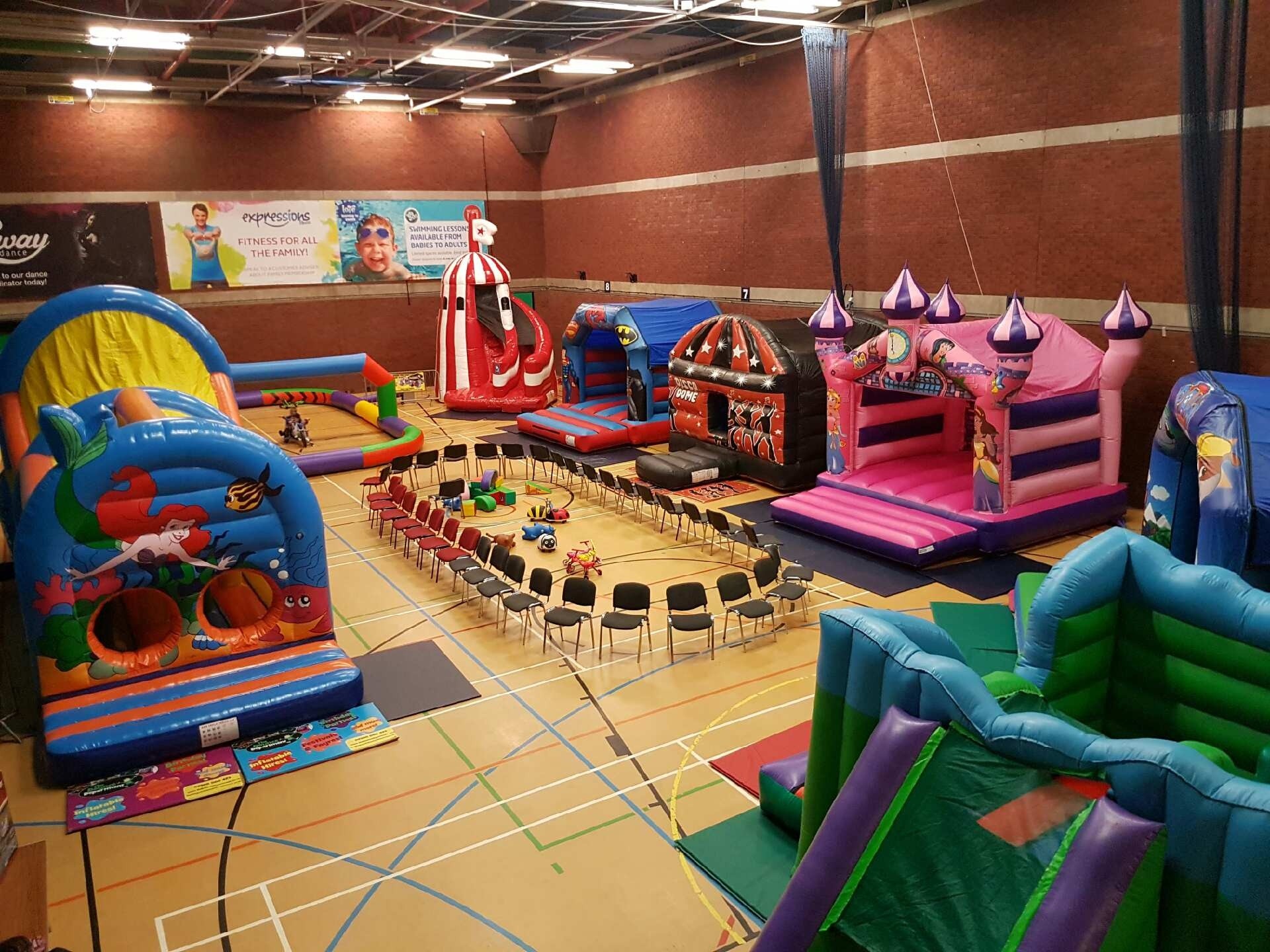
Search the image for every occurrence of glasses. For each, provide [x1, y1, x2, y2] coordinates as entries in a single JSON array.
[[358, 228, 391, 237]]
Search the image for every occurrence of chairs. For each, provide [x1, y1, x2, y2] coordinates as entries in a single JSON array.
[[356, 441, 816, 663]]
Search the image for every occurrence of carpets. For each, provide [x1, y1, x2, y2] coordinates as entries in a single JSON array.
[[727, 523, 936, 598], [484, 411, 526, 422], [476, 424, 654, 474], [353, 640, 482, 721], [672, 482, 758, 502], [426, 408, 488, 422], [719, 492, 804, 523], [925, 552, 1054, 600], [599, 475, 672, 502]]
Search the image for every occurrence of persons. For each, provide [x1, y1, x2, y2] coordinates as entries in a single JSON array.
[[185, 204, 229, 288], [279, 408, 311, 443], [344, 214, 424, 280]]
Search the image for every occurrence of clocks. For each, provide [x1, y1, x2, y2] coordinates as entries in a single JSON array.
[[885, 328, 912, 364]]
